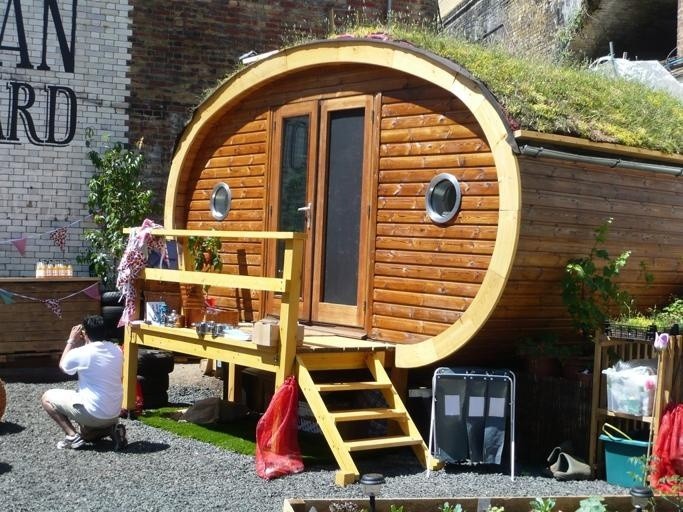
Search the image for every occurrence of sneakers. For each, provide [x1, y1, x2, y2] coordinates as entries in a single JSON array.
[[110, 423, 127, 452], [56, 432, 85, 450]]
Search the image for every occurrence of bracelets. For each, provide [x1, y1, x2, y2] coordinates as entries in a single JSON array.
[[66, 339, 79, 346]]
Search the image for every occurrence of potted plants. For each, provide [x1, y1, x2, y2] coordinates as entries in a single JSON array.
[[519, 329, 571, 377], [76, 127, 158, 338]]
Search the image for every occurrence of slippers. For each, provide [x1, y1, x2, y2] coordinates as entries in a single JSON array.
[[540, 445, 604, 480]]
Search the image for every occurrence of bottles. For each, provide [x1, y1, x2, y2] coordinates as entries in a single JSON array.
[[152, 302, 179, 326], [34, 259, 73, 277]]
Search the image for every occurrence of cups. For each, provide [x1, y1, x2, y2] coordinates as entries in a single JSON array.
[[189, 319, 223, 335], [205, 298, 215, 308]]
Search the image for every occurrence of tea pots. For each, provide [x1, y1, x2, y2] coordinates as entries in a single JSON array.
[[652, 332, 669, 351]]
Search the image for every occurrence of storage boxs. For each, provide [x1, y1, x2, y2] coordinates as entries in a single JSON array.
[[598, 370, 657, 489], [251, 321, 305, 347]]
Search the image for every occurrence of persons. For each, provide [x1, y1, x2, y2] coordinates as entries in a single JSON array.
[[40, 314, 128, 451]]
